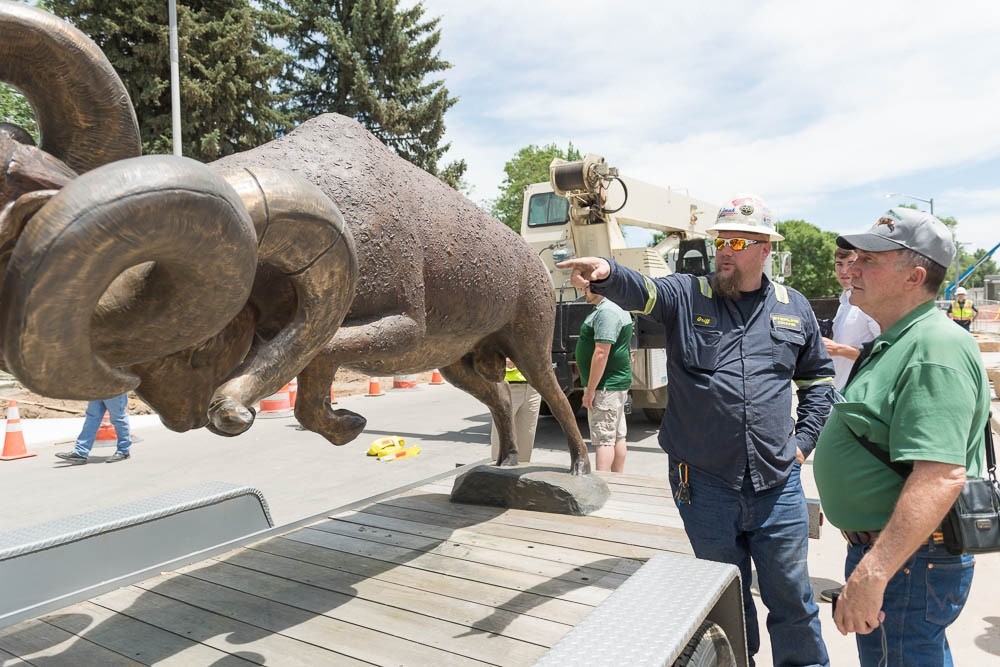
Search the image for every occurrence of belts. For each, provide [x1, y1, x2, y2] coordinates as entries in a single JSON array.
[[508, 381, 528, 384], [841, 530, 943, 547]]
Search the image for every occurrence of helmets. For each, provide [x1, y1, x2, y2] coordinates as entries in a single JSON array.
[[705, 195, 785, 241], [954, 287, 967, 295]]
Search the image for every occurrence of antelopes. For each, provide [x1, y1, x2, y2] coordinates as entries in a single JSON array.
[[0, 1, 592, 475]]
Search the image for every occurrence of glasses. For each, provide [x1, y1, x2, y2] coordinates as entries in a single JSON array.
[[714, 237, 770, 251]]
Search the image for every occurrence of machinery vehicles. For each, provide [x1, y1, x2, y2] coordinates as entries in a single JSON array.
[[494, 150, 793, 424]]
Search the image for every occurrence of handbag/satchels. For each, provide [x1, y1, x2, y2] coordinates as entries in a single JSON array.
[[941, 474, 1000, 555]]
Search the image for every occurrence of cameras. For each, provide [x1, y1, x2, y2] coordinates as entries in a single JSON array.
[[816, 318, 834, 340]]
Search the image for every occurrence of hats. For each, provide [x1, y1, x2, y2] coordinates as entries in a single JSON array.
[[837, 207, 957, 269]]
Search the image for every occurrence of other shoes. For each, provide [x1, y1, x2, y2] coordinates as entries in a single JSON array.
[[54, 450, 87, 465], [105, 453, 129, 463], [820, 585, 846, 602]]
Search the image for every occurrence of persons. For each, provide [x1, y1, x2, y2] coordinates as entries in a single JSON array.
[[554, 198, 837, 667], [54, 390, 129, 465], [576, 286, 634, 473], [490, 355, 542, 465], [813, 209, 989, 667]]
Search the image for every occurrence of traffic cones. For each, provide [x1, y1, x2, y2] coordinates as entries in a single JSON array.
[[428, 368, 445, 385], [91, 405, 145, 448], [364, 376, 385, 397], [287, 375, 298, 409], [391, 374, 417, 390], [0, 399, 37, 461]]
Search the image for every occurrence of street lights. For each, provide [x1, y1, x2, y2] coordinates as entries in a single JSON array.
[[884, 191, 934, 215]]
